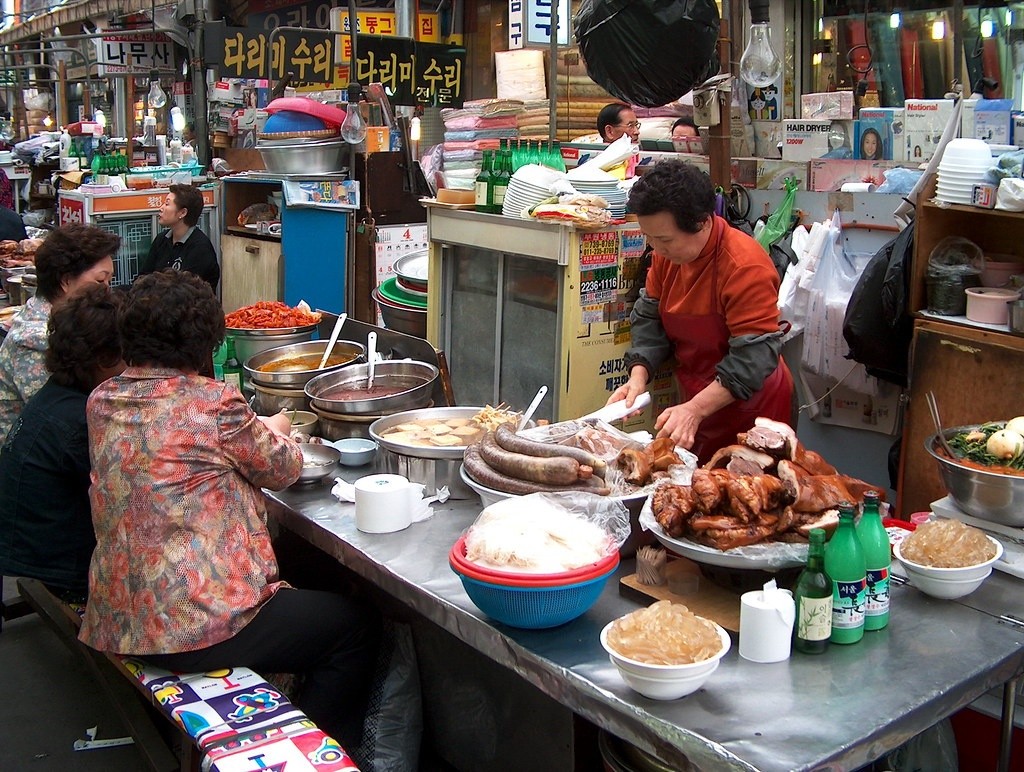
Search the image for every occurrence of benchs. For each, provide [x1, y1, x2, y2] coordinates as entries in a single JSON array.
[[0, 577, 359, 772]]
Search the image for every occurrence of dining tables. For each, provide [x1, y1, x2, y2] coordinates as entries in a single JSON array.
[[261, 455, 1024, 772]]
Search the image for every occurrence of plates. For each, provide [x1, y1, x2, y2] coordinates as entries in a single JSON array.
[[501, 163, 629, 224], [245, 224, 257, 229], [392, 247, 428, 280]]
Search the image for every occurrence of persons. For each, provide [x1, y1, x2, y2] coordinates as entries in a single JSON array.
[[596, 104, 642, 147], [820, 120, 854, 159], [0, 280, 126, 590], [915, 146, 921, 157], [78, 269, 373, 740], [0, 224, 121, 446], [602, 160, 798, 474], [860, 128, 883, 160], [140, 184, 220, 379], [671, 117, 700, 138]]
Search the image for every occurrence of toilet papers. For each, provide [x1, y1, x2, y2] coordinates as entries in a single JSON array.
[[155, 134, 167, 165], [394, 0, 415, 39], [737, 577, 796, 663], [840, 182, 876, 193], [143, 116, 156, 145], [353, 473, 433, 534]]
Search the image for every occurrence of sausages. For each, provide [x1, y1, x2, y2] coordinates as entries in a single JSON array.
[[463, 421, 611, 495]]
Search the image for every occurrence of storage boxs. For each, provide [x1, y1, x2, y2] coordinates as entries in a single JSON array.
[[731, 91, 1024, 192]]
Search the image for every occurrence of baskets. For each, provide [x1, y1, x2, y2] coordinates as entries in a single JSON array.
[[448, 529, 620, 629], [256, 99, 345, 137]]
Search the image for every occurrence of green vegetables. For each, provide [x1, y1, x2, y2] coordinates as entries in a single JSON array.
[[944, 423, 1024, 471]]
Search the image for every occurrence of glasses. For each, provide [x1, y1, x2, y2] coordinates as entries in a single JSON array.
[[611, 123, 641, 132]]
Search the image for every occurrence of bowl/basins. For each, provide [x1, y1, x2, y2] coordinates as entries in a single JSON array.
[[304, 359, 440, 414], [254, 137, 346, 173], [371, 275, 427, 341], [242, 339, 367, 389], [256, 410, 377, 484], [893, 422, 1023, 599], [933, 136, 1023, 334], [600, 616, 732, 700], [460, 446, 697, 561], [235, 328, 318, 376], [697, 560, 805, 593], [0, 264, 38, 306], [366, 407, 536, 459]]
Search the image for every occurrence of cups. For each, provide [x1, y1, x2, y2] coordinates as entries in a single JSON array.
[[256, 212, 270, 233], [144, 122, 192, 166]]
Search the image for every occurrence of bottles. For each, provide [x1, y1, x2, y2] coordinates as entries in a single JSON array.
[[222, 335, 244, 398], [59, 127, 129, 184], [856, 489, 891, 631], [791, 527, 832, 654], [474, 135, 566, 217], [824, 500, 867, 644]]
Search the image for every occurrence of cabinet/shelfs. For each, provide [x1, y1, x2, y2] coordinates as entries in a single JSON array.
[[896, 173, 1024, 524], [220, 152, 433, 326]]
